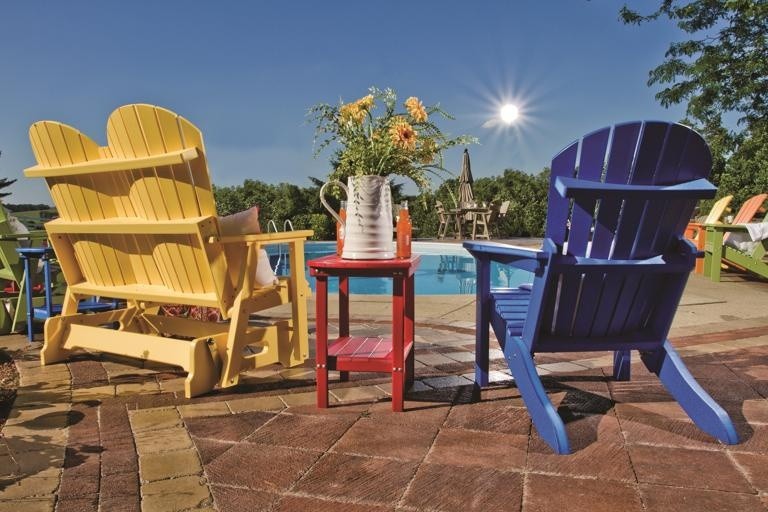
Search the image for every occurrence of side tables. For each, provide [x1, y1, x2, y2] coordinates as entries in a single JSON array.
[[14, 247, 120, 343], [305, 252, 425, 414]]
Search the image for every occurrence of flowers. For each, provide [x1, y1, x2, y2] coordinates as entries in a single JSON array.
[[301, 88, 484, 209]]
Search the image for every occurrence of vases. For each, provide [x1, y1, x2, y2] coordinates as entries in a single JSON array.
[[319, 175, 396, 260]]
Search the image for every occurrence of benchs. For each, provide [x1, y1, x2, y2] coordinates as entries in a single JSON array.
[[21, 101, 316, 400]]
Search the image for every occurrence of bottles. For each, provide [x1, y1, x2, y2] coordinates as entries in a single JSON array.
[[336, 201, 347, 256], [394, 198, 413, 259]]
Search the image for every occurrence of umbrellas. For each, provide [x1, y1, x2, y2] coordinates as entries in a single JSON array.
[[456, 148, 476, 206]]
